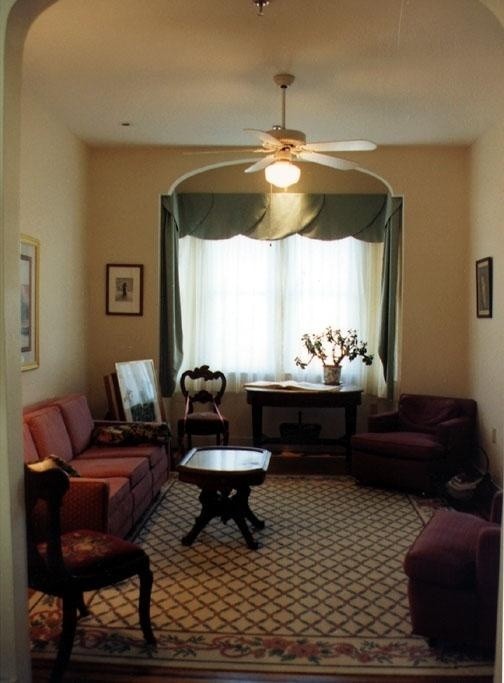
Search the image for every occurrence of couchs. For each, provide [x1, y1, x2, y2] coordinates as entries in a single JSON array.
[[22, 396, 175, 545]]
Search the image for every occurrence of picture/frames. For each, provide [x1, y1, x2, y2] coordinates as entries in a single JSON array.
[[106, 263, 144, 315], [475, 258, 493, 318], [115, 359, 166, 425], [22, 233, 40, 371]]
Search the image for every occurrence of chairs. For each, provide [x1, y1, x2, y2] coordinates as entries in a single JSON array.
[[177, 365, 229, 451], [25, 468, 155, 683], [352, 393, 477, 494], [402, 492, 502, 652], [103, 373, 124, 421]]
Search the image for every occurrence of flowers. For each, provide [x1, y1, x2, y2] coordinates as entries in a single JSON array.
[[294, 326, 374, 371]]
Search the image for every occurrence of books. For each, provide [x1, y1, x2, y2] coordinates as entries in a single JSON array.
[[243, 380, 339, 393]]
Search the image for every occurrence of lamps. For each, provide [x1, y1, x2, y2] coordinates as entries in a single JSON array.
[[266, 159, 300, 189]]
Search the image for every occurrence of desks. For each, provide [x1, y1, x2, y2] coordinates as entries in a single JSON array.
[[244, 384, 363, 473]]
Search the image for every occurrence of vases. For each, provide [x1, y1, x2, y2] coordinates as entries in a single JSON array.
[[324, 364, 341, 385]]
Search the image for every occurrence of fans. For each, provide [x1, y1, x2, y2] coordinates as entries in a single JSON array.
[[185, 74, 377, 173]]
